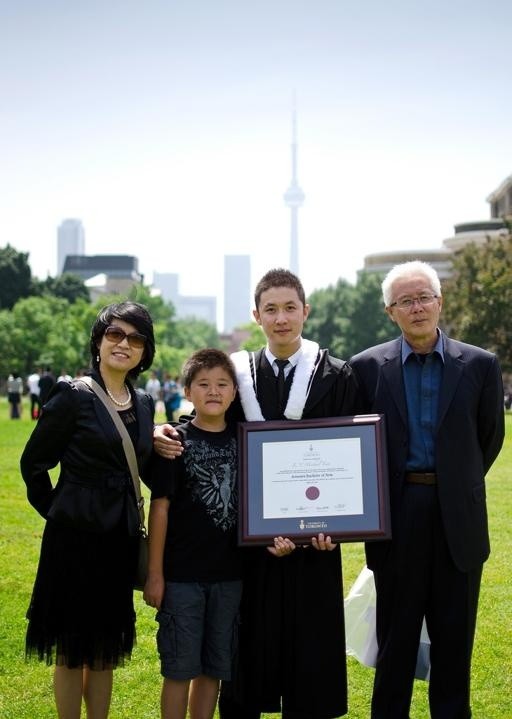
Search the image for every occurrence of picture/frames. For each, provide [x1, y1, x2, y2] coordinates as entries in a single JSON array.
[[237, 412, 393, 547]]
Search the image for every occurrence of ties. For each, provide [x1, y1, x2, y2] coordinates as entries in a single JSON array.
[[269, 359, 290, 421]]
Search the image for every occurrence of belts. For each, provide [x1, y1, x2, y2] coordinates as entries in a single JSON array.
[[404, 471, 437, 485]]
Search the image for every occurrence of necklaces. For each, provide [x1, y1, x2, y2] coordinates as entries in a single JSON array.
[[104, 383, 133, 407]]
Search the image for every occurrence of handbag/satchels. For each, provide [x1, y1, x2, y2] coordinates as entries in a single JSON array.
[[168, 393, 178, 403], [132, 531, 149, 589]]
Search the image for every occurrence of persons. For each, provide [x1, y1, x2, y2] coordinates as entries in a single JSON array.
[[143, 348, 297, 719], [6, 366, 87, 419], [155, 269, 363, 718], [19, 300, 152, 718], [132, 372, 186, 423], [347, 261, 505, 719]]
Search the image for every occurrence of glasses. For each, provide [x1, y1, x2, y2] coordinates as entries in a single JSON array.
[[388, 294, 439, 308], [101, 326, 149, 349]]
